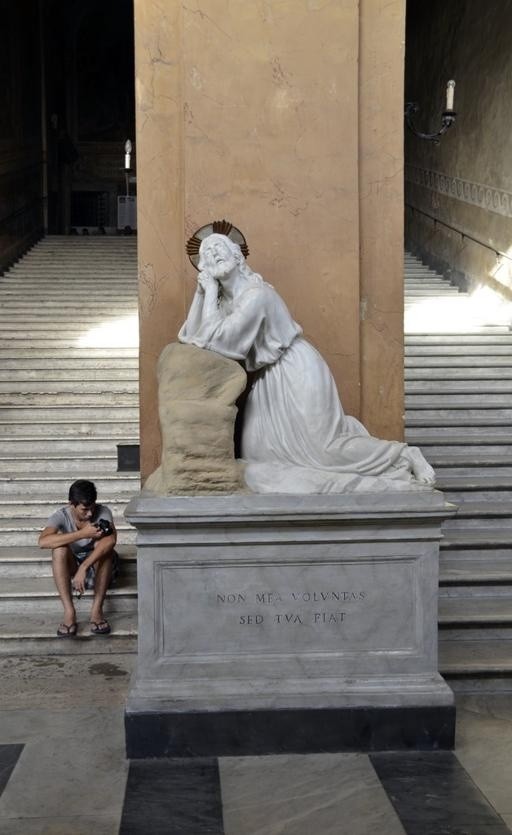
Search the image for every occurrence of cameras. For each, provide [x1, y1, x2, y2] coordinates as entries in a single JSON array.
[[96, 519, 113, 536]]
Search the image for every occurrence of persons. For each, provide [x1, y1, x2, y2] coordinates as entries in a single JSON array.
[[37, 479, 122, 639], [176, 231, 437, 494]]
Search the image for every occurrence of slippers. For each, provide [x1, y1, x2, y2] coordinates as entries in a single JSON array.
[[57, 623, 77, 635], [90, 619, 110, 634]]
